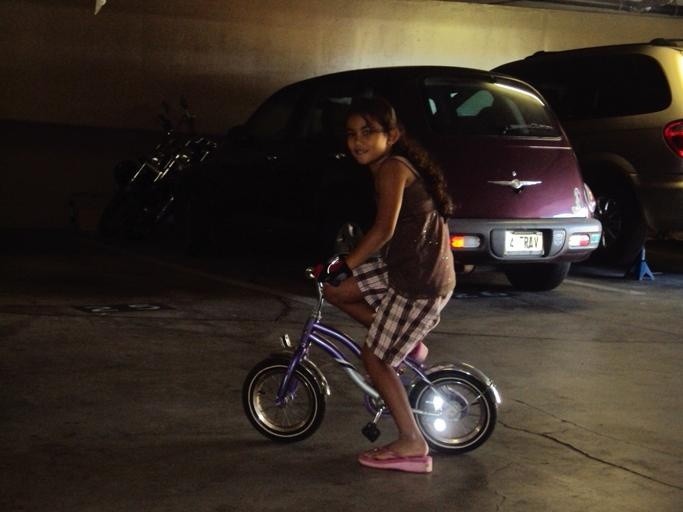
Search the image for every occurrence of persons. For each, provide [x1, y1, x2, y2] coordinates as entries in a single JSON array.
[[313, 95, 458, 474]]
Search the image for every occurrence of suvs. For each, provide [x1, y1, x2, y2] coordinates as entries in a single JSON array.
[[483, 34, 682, 281]]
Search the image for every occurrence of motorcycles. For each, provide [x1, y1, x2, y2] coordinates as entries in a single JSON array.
[[95, 97, 218, 254]]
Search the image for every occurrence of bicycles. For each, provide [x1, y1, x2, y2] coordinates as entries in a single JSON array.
[[237, 256, 503, 460]]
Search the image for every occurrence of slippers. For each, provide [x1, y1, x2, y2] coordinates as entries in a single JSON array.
[[398, 341, 430, 363], [357, 444, 434, 477]]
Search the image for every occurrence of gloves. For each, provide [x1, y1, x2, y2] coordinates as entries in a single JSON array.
[[312, 257, 353, 287]]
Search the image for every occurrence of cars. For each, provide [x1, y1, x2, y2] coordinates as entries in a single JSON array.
[[205, 55, 607, 299]]
